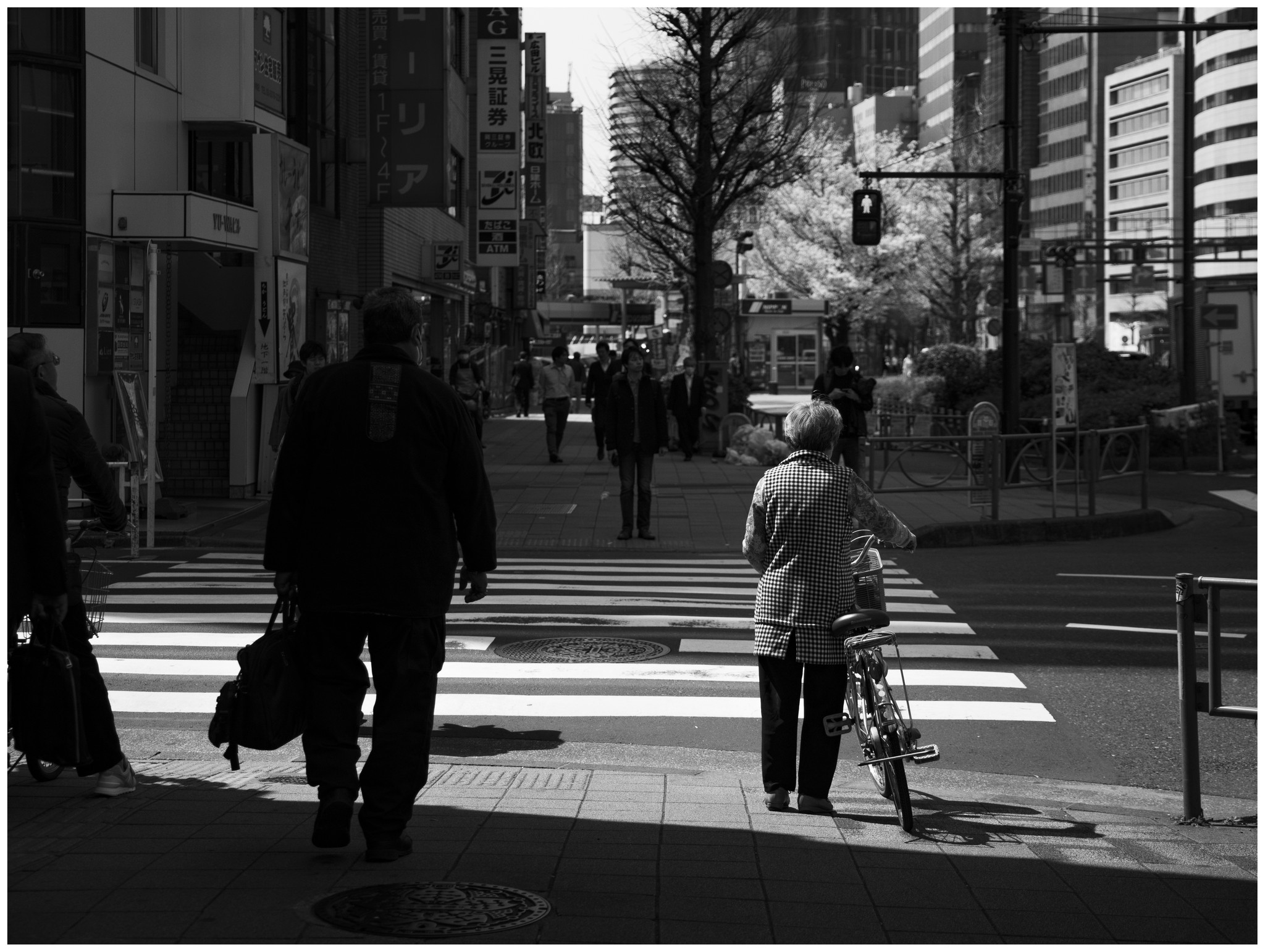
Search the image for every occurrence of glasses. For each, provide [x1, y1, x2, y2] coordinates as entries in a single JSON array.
[[34, 355, 60, 377]]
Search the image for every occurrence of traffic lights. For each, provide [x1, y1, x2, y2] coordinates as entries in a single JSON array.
[[852, 189, 882, 245], [1045, 246, 1077, 258]]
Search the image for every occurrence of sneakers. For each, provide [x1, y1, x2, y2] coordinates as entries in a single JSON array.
[[94, 751, 137, 797]]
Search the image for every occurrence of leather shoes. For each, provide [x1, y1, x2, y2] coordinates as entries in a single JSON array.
[[799, 794, 832, 814], [764, 790, 791, 810]]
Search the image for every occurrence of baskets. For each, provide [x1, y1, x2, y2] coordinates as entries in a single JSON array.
[[849, 548, 886, 612], [19, 556, 113, 635]]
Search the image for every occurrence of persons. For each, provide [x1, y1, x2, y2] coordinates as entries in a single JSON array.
[[812, 345, 873, 475], [610, 350, 616, 360], [260, 287, 497, 862], [269, 341, 328, 452], [449, 350, 488, 448], [742, 399, 918, 813], [728, 353, 738, 368], [539, 346, 575, 462], [569, 351, 585, 412], [586, 341, 616, 460], [607, 339, 654, 383], [7, 331, 137, 796], [510, 353, 534, 417], [605, 347, 667, 539], [671, 356, 704, 461]]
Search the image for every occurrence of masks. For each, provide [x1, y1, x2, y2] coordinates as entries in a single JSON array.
[[686, 367, 694, 375]]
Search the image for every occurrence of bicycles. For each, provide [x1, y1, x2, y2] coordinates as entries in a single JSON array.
[[7, 515, 137, 783], [830, 527, 941, 831]]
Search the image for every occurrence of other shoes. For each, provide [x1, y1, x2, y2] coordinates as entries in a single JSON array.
[[639, 530, 655, 539], [366, 836, 412, 862], [550, 456, 562, 463], [618, 531, 632, 539], [517, 410, 521, 418], [312, 784, 352, 848], [598, 447, 604, 459], [685, 456, 691, 461]]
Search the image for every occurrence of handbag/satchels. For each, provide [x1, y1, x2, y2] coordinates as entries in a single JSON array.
[[208, 589, 371, 751], [14, 614, 94, 767]]
